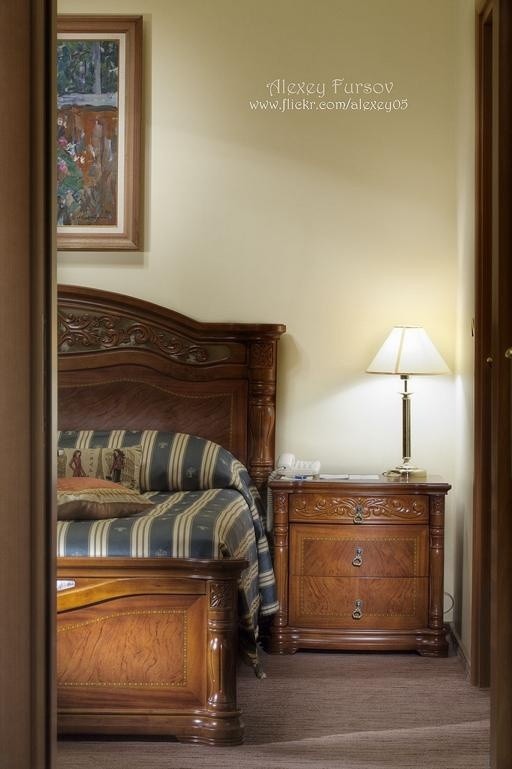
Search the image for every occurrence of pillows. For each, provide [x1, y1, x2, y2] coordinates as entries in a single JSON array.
[[57, 477, 155, 520], [63, 444, 143, 492], [57, 428, 249, 492]]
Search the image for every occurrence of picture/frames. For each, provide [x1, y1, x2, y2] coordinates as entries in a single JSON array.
[[55, 13, 145, 251]]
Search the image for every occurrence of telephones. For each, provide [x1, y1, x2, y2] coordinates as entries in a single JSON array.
[[276, 452, 322, 476]]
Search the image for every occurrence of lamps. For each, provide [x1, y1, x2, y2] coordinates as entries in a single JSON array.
[[364, 325, 452, 482]]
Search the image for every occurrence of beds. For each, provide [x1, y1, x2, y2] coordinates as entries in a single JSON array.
[[55, 283, 286, 747]]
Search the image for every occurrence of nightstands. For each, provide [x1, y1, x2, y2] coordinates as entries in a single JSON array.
[[269, 479, 452, 658]]
